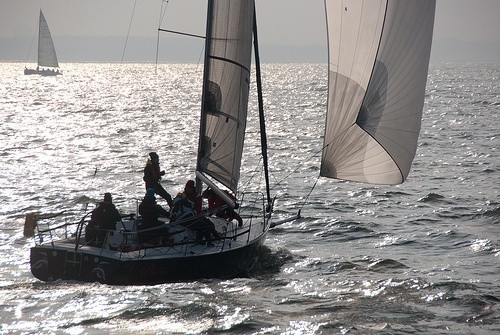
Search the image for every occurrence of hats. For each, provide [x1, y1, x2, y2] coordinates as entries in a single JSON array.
[[187, 180, 194, 187], [147, 188, 154, 195], [149, 152, 159, 160]]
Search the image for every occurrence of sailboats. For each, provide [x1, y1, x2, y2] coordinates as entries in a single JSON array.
[[31, 1, 439, 281], [23, 9, 64, 75]]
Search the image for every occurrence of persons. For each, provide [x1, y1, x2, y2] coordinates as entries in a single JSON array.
[[139, 189, 170, 227], [171, 180, 243, 247], [85, 193, 122, 247], [142, 151, 173, 209]]
